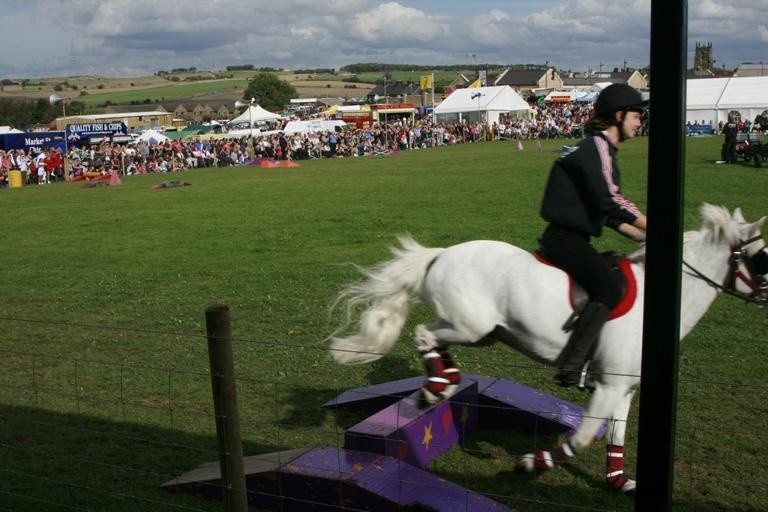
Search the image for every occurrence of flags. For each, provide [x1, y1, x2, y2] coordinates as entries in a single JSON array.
[[467, 79, 480, 88], [420, 75, 432, 91]]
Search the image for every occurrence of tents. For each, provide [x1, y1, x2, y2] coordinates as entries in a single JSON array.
[[283, 120, 347, 136], [228, 97, 277, 127], [128, 129, 170, 145], [258, 130, 283, 137], [161, 129, 206, 141], [685, 76, 768, 136], [228, 129, 261, 139], [433, 85, 531, 124], [182, 125, 210, 133]]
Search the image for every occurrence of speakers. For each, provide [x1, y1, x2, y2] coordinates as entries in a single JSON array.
[[557, 301, 609, 387]]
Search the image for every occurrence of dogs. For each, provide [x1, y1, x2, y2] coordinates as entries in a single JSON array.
[[321, 201, 768, 494]]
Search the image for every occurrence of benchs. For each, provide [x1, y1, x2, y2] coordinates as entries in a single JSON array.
[[420, 75, 432, 91], [467, 79, 480, 88]]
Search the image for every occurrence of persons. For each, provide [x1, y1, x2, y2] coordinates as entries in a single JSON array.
[[539, 83, 650, 387], [0, 102, 650, 185], [686, 110, 768, 164]]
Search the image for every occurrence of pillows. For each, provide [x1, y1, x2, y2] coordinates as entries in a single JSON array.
[[596, 84, 649, 115]]
